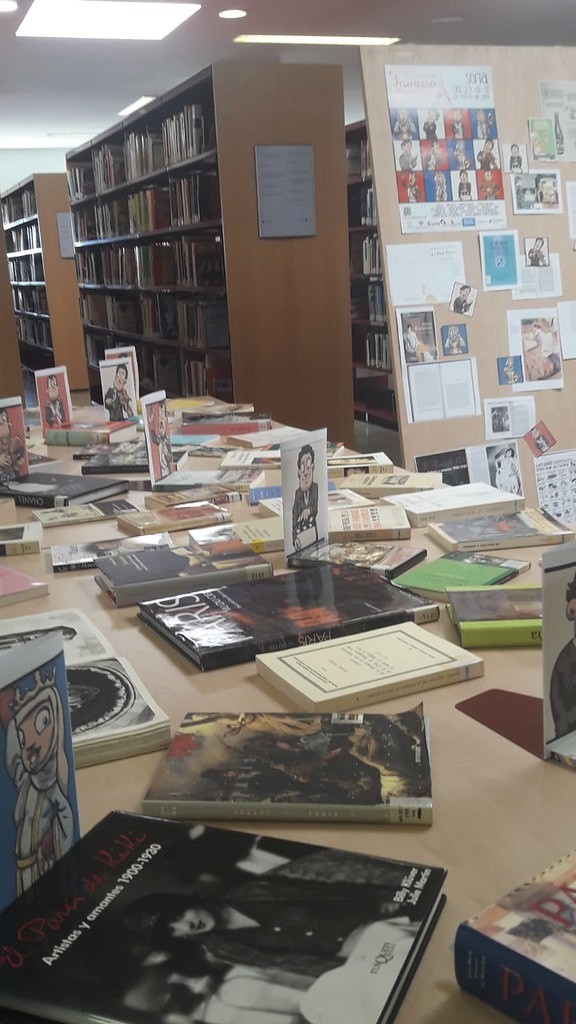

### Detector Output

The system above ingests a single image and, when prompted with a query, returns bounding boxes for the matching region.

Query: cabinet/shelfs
[0,59,398,454]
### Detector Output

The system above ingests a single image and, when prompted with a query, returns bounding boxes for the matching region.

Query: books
[1,103,394,400]
[0,403,576,1024]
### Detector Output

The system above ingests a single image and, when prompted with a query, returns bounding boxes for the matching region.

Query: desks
[0,395,575,1024]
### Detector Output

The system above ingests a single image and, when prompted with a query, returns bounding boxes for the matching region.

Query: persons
[404,325,419,355]
[494,448,519,494]
[122,872,422,977]
